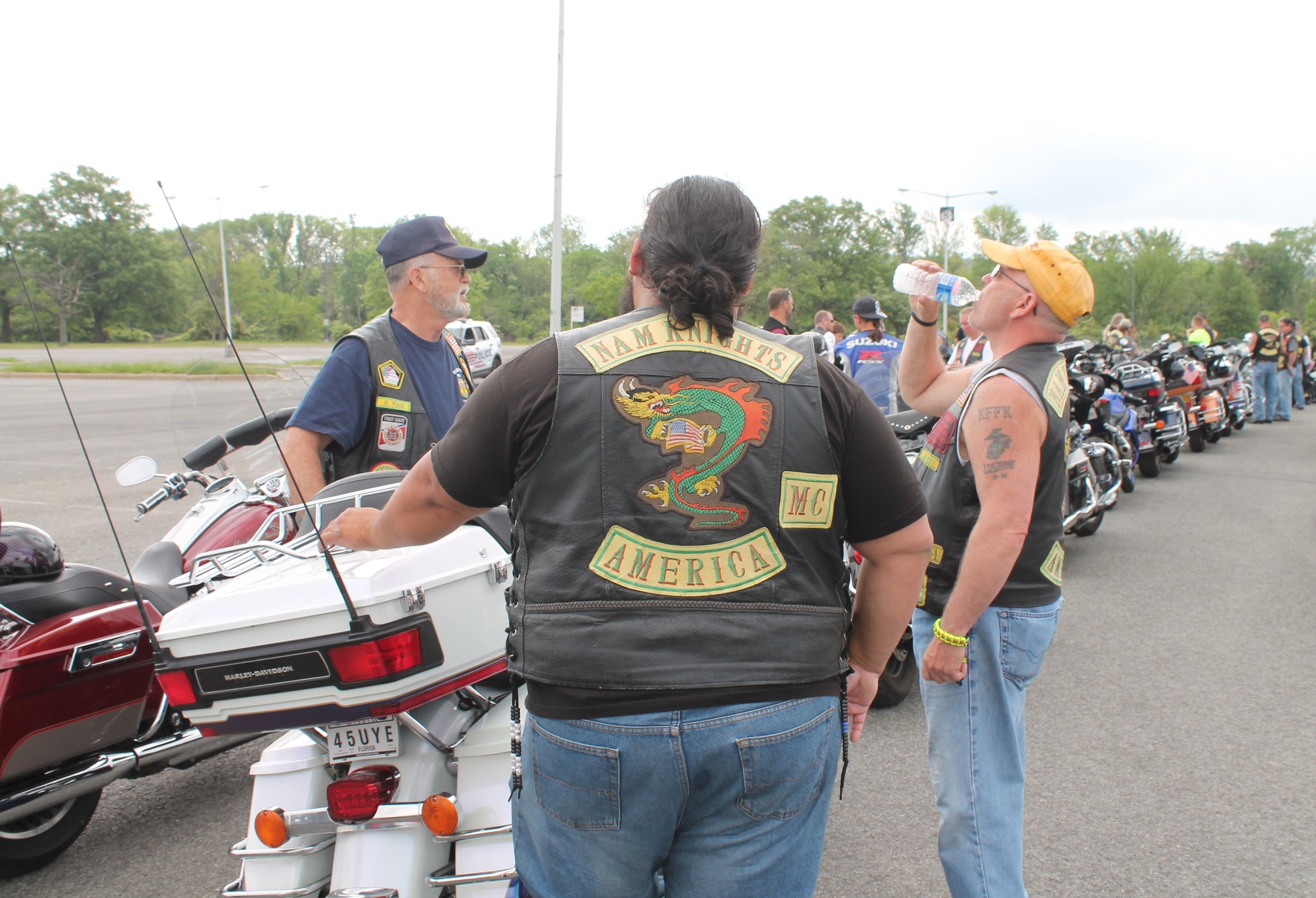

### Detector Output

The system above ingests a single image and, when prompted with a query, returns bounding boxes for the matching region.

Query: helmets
[0,522,64,580]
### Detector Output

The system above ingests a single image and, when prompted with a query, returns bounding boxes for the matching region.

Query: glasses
[410,264,465,285]
[821,319,834,323]
[783,290,792,305]
[990,264,1037,316]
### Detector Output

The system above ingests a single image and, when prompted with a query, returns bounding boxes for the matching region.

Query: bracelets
[932,618,969,647]
[911,309,937,326]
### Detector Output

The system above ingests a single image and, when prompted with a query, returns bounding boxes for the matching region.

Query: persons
[835,296,913,416]
[801,331,829,361]
[810,310,835,364]
[935,307,993,370]
[1103,313,1140,354]
[1274,316,1316,421]
[762,287,794,335]
[1247,315,1280,423]
[318,175,932,898]
[833,320,844,344]
[1186,313,1218,349]
[898,239,1094,898]
[280,216,488,518]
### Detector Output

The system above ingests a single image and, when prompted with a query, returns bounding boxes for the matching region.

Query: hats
[1260,314,1269,321]
[982,238,1095,327]
[375,217,488,270]
[852,296,888,319]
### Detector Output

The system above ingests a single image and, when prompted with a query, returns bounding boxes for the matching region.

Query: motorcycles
[13,177,524,898]
[833,326,1260,712]
[0,346,316,878]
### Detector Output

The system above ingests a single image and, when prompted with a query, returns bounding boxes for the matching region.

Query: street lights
[897,186,998,335]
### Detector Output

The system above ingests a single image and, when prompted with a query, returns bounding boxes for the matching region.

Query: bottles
[893,263,981,307]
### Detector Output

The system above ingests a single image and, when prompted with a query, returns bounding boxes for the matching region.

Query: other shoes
[1248,419,1264,424]
[1274,416,1289,422]
[1294,405,1303,410]
[1264,419,1272,423]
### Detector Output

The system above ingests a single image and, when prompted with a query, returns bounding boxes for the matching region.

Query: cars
[442,318,505,378]
